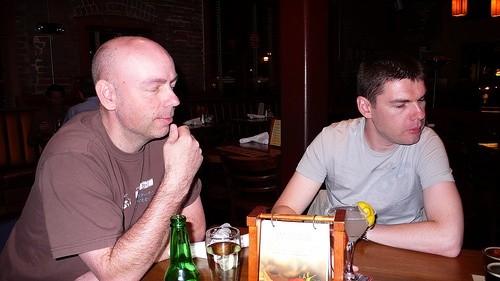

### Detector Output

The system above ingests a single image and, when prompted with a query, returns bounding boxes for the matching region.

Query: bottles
[164,215,201,281]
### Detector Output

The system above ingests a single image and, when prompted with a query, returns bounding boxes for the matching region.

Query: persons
[0,36,206,281]
[270,52,464,257]
[27,84,71,146]
[64,75,101,123]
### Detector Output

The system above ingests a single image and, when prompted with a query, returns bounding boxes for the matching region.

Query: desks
[214,140,280,195]
[141,227,486,281]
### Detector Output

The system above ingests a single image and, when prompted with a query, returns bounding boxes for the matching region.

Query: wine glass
[325,206,376,281]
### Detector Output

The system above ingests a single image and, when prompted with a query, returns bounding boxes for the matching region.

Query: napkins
[168,233,248,260]
[240,131,270,145]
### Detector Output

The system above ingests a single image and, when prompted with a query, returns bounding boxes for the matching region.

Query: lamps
[491,0,500,17]
[452,0,467,17]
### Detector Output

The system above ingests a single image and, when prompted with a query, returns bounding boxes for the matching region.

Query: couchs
[0,108,54,214]
[190,99,258,145]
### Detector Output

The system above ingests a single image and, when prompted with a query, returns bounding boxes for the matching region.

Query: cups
[485,263,500,281]
[484,247,500,271]
[206,227,241,281]
